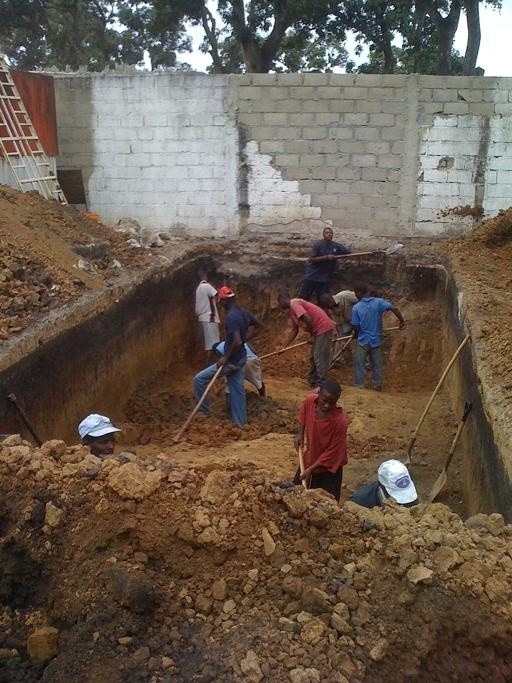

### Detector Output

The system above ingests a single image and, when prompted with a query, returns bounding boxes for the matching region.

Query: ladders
[0,58,68,206]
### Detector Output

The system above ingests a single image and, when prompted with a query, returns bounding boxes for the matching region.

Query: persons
[77,413,123,456]
[274,291,338,395]
[194,267,221,368]
[320,288,370,368]
[296,225,375,332]
[348,282,407,391]
[290,375,350,505]
[211,338,267,417]
[189,285,266,426]
[349,458,420,512]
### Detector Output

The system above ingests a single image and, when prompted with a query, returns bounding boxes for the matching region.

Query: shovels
[404,334,472,469]
[332,243,405,258]
[428,400,472,503]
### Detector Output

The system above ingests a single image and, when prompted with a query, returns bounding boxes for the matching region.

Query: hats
[217,285,236,301]
[78,412,121,440]
[376,458,419,506]
